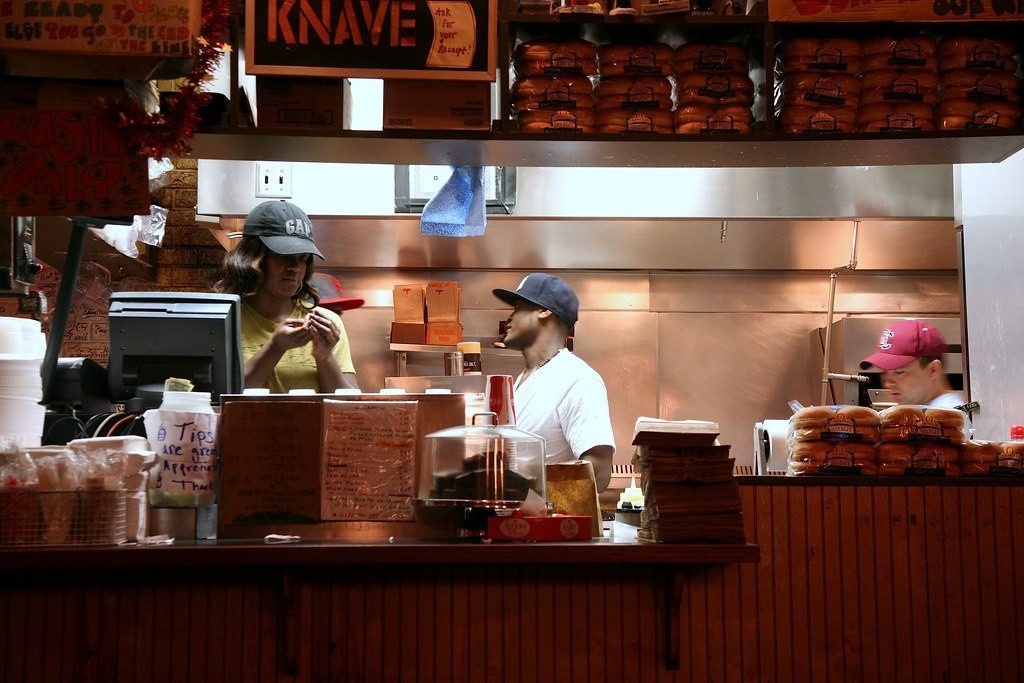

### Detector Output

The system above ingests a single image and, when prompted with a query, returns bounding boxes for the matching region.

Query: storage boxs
[256,74,352,132]
[149,505,218,540]
[391,321,426,345]
[382,78,490,130]
[425,321,462,345]
[0,489,127,548]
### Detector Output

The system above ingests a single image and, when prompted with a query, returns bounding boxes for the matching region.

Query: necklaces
[534,351,559,371]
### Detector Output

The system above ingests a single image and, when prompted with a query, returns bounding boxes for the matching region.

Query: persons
[860,318,971,420]
[223,202,358,393]
[492,272,616,493]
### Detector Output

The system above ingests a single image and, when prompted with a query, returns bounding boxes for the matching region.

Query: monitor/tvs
[107,292,244,405]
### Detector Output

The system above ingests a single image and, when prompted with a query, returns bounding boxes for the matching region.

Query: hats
[858,320,946,370]
[492,272,579,328]
[243,199,326,262]
[307,273,365,310]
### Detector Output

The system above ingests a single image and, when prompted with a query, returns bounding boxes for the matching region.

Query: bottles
[615,474,645,509]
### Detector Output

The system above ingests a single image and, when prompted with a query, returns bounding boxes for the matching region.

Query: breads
[789,407,1024,474]
[510,38,1021,129]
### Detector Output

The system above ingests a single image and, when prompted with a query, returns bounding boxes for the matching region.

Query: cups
[444,351,465,376]
[482,374,518,471]
